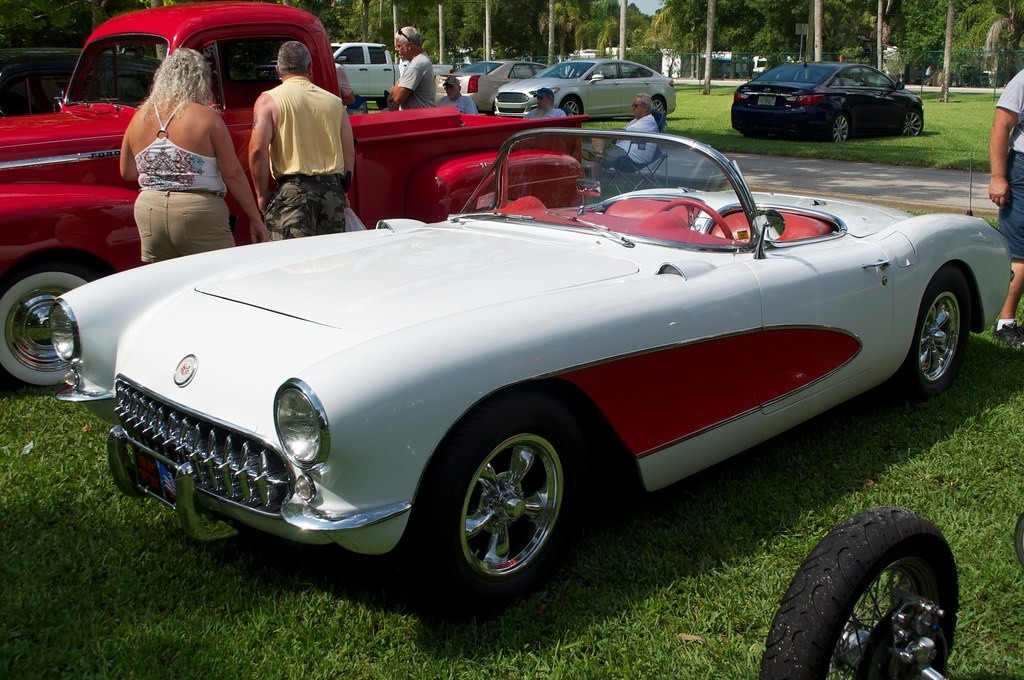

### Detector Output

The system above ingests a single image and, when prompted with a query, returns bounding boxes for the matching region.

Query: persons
[120,48,269,263]
[250,41,357,240]
[334,62,356,105]
[925,66,933,85]
[390,26,437,110]
[580,93,660,182]
[381,94,399,112]
[437,76,479,115]
[988,68,1024,348]
[523,88,566,119]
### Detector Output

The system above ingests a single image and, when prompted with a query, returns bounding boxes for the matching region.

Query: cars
[433,59,566,117]
[0,46,164,119]
[493,57,677,125]
[730,60,926,144]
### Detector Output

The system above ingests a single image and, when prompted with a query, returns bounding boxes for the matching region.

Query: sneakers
[992,322,1024,348]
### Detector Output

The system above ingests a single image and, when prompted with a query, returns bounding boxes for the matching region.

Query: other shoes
[582,148,606,164]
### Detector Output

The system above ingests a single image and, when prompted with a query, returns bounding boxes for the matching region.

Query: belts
[279,174,338,182]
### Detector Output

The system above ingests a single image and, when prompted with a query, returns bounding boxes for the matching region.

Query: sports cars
[46,123,1016,615]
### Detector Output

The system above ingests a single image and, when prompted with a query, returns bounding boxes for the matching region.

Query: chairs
[711,211,832,242]
[603,198,704,229]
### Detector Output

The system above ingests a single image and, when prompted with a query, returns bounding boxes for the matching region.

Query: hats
[533,88,554,98]
[443,77,460,85]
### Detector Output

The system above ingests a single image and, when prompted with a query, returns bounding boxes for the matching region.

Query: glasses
[632,104,641,108]
[536,96,544,100]
[446,84,454,89]
[398,27,410,43]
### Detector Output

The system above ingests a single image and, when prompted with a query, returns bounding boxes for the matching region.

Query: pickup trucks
[330,41,454,111]
[0,0,602,390]
